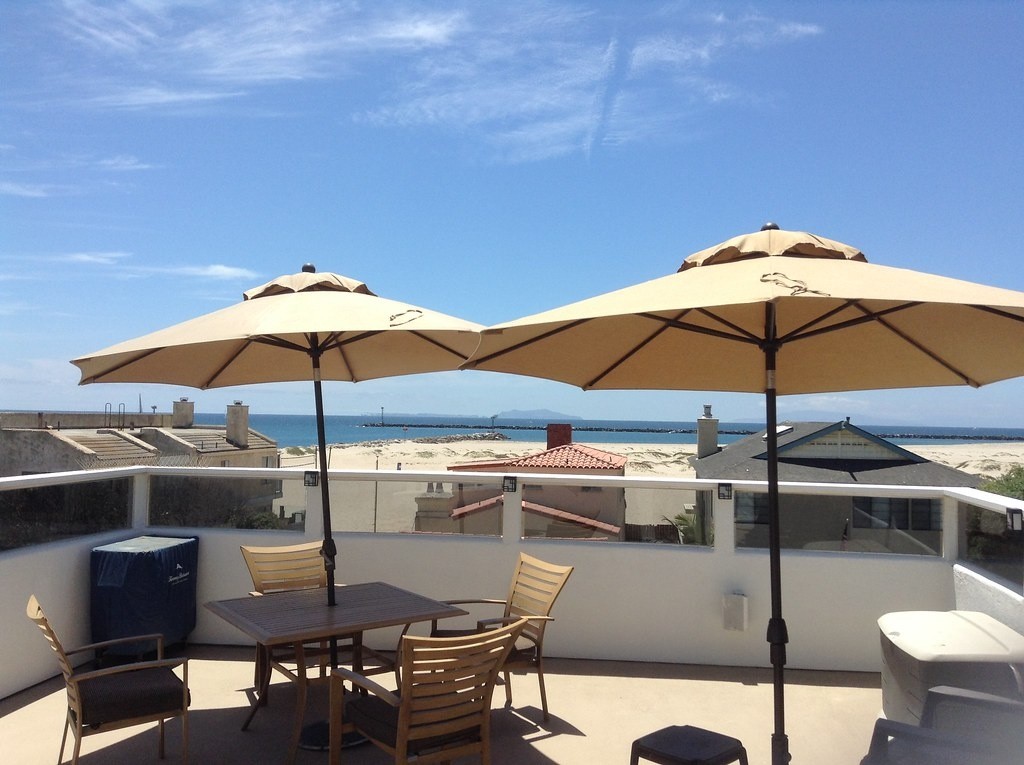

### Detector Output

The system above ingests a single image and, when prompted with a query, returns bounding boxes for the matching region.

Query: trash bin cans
[396,462,402,470]
[873,607,1024,734]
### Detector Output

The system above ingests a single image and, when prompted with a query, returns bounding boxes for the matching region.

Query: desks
[203,582,468,765]
[90,537,199,668]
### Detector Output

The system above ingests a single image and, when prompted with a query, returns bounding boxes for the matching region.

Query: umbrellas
[68,263,489,670]
[459,222,1024,765]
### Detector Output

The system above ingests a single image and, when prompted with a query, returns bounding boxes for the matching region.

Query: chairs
[429,552,574,721]
[328,618,530,765]
[240,541,358,705]
[26,594,189,765]
[860,685,1024,765]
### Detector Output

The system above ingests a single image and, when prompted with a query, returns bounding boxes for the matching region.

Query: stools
[629,725,753,765]
